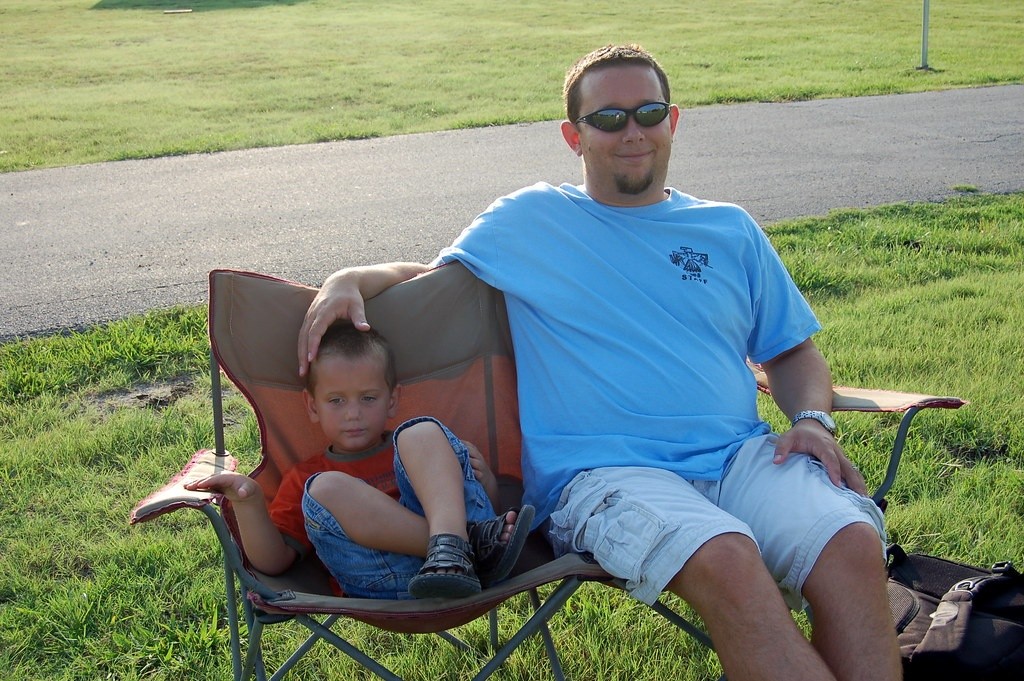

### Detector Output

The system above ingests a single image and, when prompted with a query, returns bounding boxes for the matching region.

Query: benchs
[130,259,967,679]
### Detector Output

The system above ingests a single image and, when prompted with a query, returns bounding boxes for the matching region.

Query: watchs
[792,410,836,437]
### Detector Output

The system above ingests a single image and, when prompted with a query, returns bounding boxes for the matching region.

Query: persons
[184,318,536,599]
[298,46,902,681]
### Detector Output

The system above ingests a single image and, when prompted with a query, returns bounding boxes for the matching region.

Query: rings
[852,465,856,470]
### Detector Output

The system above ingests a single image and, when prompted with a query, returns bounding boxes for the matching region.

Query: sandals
[408,533,481,600]
[466,504,536,582]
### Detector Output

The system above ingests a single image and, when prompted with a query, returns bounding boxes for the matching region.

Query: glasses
[575,102,670,132]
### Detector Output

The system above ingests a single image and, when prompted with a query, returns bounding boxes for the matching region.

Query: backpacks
[881,541,1024,681]
